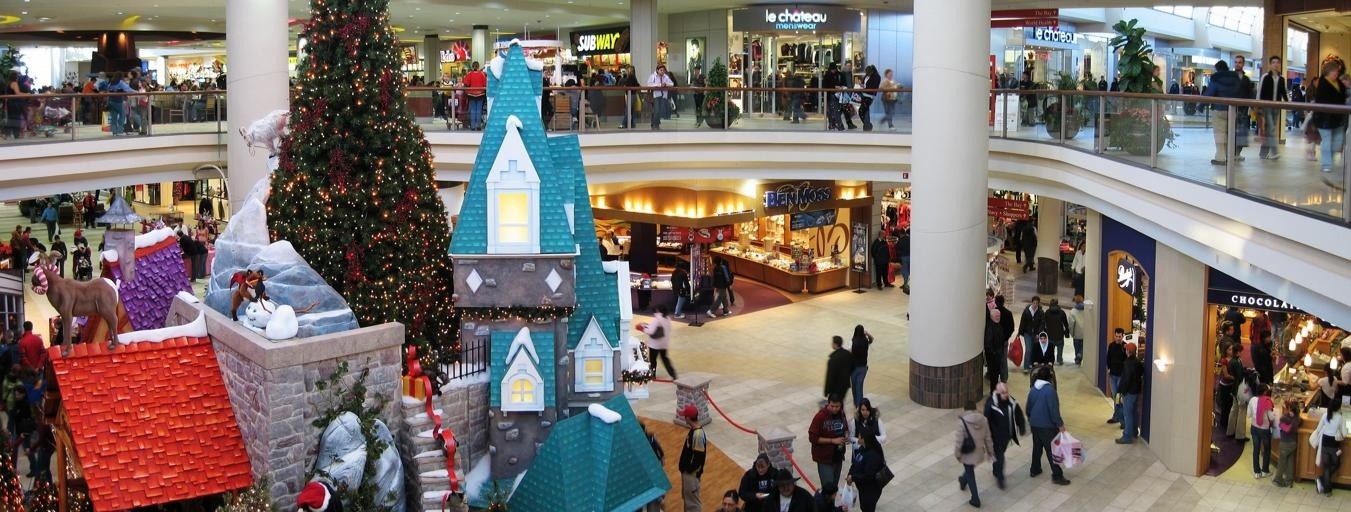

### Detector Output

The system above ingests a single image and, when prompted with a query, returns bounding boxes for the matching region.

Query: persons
[1115,342,1145,444]
[1218,306,1351,497]
[1104,327,1128,430]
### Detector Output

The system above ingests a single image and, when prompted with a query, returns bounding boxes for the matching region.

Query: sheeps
[238,109,291,158]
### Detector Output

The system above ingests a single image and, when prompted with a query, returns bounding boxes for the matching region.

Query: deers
[30,248,119,356]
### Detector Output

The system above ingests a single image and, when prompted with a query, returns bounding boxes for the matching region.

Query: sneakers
[1052,475,1069,485]
[706,310,715,317]
[1030,468,1042,476]
[674,312,685,319]
[724,310,731,315]
[1254,469,1290,487]
[969,499,979,507]
[1107,418,1138,443]
[957,475,966,490]
[1314,475,1322,493]
[878,283,905,291]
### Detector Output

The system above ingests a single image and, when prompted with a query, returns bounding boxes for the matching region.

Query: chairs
[51,83,218,125]
[576,101,602,131]
[552,94,574,132]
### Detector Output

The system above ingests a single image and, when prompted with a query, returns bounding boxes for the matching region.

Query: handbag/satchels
[1007,336,1024,367]
[1335,429,1344,441]
[957,414,975,453]
[874,464,894,489]
[1268,420,1274,433]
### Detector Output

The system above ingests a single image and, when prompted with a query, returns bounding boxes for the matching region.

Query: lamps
[1152,358,1169,372]
[1288,312,1339,371]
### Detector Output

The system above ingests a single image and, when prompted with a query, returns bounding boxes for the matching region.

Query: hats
[771,468,800,483]
[296,481,333,512]
[1123,342,1137,352]
[679,406,698,416]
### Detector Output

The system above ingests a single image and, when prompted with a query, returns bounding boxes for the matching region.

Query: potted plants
[1104,18,1180,155]
[698,55,742,129]
[1044,73,1086,141]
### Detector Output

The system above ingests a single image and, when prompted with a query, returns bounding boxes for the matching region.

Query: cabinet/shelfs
[593,234,851,314]
